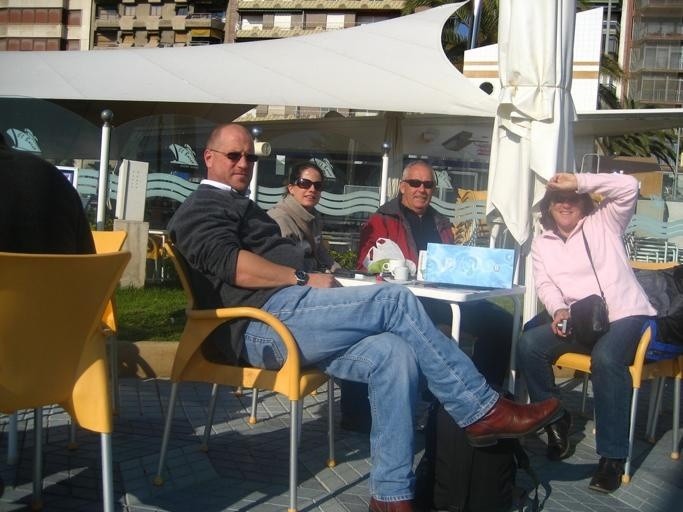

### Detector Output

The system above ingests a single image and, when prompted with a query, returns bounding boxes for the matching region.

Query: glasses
[210,149,259,161]
[402,180,435,188]
[294,179,324,190]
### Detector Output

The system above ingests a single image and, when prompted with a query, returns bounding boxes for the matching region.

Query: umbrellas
[486,0,582,332]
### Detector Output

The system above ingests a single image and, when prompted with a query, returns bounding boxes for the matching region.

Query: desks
[332,273,525,397]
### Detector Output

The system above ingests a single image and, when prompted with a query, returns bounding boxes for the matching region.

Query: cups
[354,272,364,279]
[391,266,409,282]
[382,259,405,276]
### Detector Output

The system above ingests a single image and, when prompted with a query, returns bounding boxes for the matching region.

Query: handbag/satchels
[571,295,609,342]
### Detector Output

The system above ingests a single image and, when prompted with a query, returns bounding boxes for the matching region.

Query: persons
[0,136,99,256]
[357,157,513,393]
[265,162,349,269]
[515,170,659,495]
[164,122,567,508]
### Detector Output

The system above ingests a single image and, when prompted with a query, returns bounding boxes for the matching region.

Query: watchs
[294,266,309,287]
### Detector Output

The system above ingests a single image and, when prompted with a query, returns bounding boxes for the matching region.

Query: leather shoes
[589,457,625,493]
[369,496,415,512]
[464,395,570,460]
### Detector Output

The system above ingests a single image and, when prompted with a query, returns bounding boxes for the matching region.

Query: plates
[379,276,419,286]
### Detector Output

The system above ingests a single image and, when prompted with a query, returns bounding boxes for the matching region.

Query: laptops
[57,165,79,191]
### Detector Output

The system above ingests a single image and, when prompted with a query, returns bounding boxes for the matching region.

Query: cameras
[556,319,568,334]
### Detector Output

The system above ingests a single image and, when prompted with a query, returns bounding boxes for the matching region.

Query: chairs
[0,251,131,512]
[552,260,683,483]
[91,230,129,415]
[154,244,335,511]
